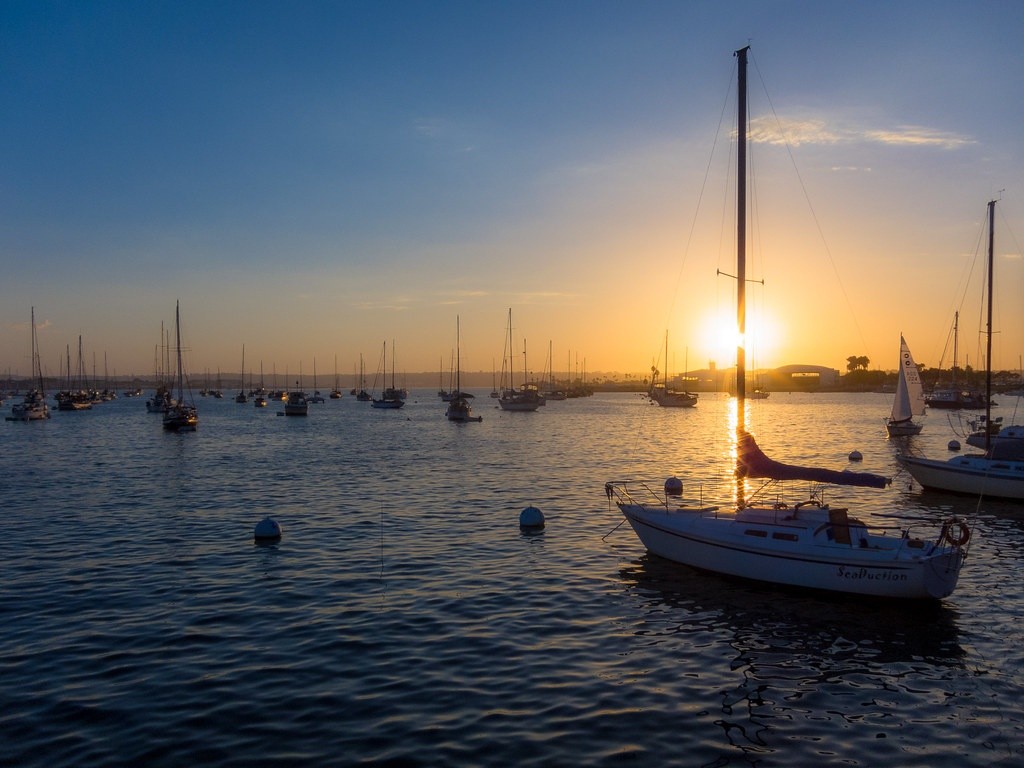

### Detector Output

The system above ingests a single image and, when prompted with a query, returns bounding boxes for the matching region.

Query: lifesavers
[942,517,970,546]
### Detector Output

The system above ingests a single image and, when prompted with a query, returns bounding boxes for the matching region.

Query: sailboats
[0,304,142,421]
[588,40,983,602]
[236,343,325,415]
[437,314,485,422]
[199,366,224,399]
[357,352,374,401]
[885,330,928,438]
[370,338,408,409]
[146,328,178,413]
[726,347,771,400]
[351,361,359,396]
[491,307,593,410]
[638,324,700,407]
[162,298,200,431]
[329,354,343,399]
[896,199,1024,501]
